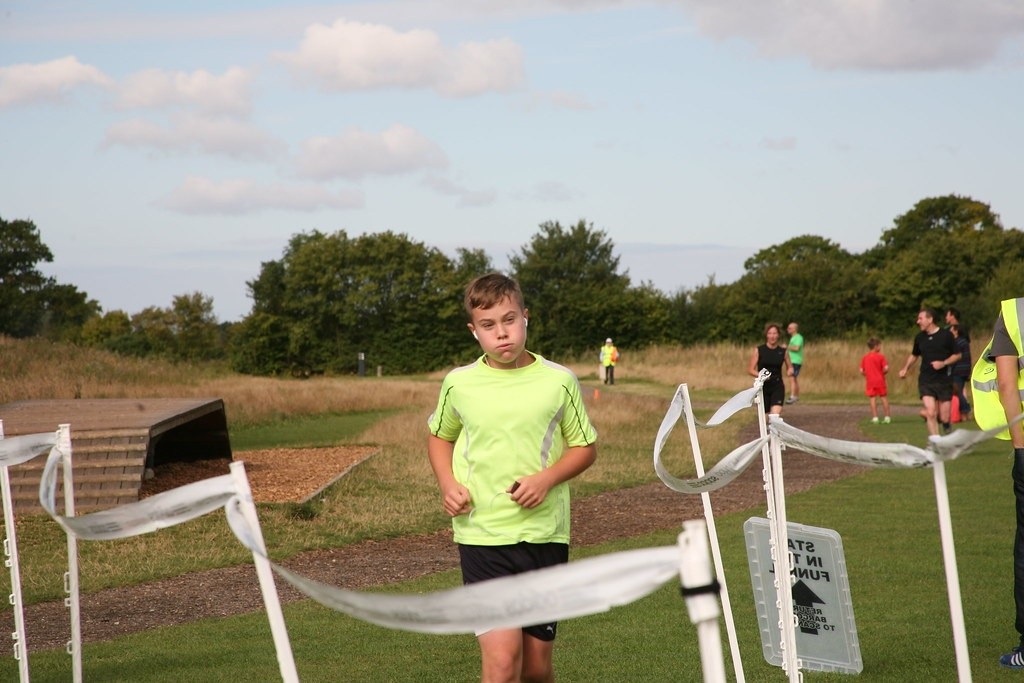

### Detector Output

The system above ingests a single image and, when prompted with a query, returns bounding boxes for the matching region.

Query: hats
[605,338,613,342]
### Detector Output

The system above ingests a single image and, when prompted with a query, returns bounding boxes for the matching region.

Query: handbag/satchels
[598,362,605,380]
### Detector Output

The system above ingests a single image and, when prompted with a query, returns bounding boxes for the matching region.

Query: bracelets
[943,361,947,366]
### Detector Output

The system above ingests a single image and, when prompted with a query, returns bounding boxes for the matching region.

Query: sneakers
[868,415,891,424]
[785,396,799,404]
[1000,646,1023,669]
[941,421,952,432]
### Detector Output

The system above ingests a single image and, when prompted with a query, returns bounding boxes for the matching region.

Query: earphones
[473,330,479,341]
[523,317,528,328]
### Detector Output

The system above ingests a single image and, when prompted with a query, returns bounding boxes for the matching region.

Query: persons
[428,272,599,683]
[781,321,804,404]
[750,322,795,425]
[898,309,964,445]
[970,297,1023,668]
[942,309,971,401]
[599,337,618,386]
[859,336,893,425]
[948,324,974,422]
